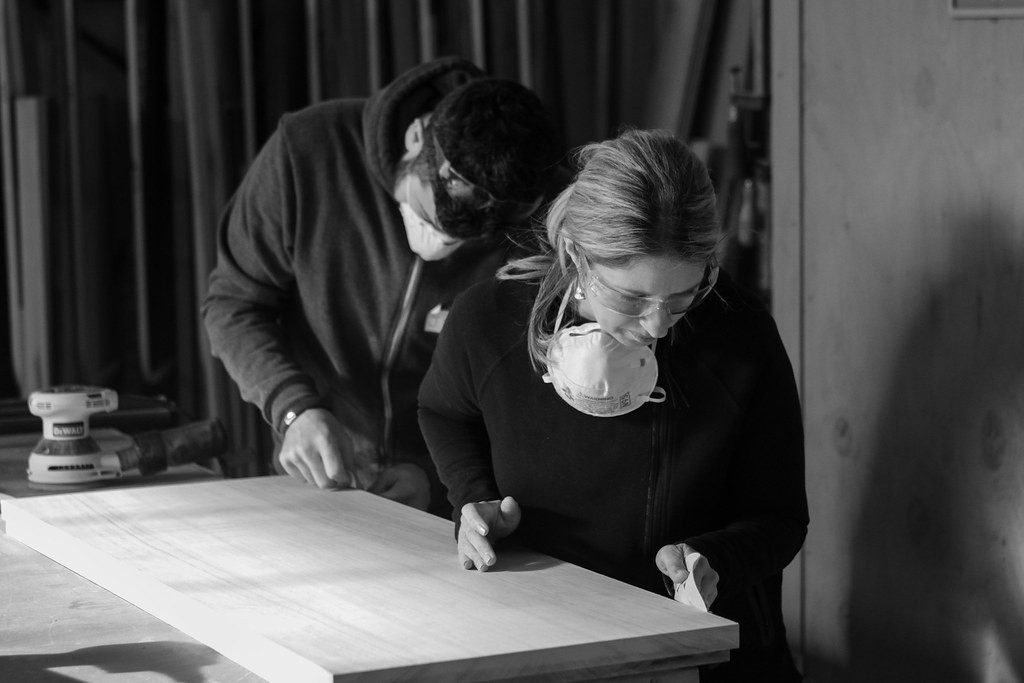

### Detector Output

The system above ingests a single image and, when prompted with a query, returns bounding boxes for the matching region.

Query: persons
[415,126,810,683]
[200,55,572,525]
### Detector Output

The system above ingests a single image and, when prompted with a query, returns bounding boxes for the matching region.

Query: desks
[0,451,731,683]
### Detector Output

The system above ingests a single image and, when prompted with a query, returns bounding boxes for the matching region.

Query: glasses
[432,135,544,220]
[581,254,719,318]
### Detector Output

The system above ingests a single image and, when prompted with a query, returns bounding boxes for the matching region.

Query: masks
[398,171,465,261]
[542,282,667,417]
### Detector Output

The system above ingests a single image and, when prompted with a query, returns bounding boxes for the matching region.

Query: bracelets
[279,401,339,433]
